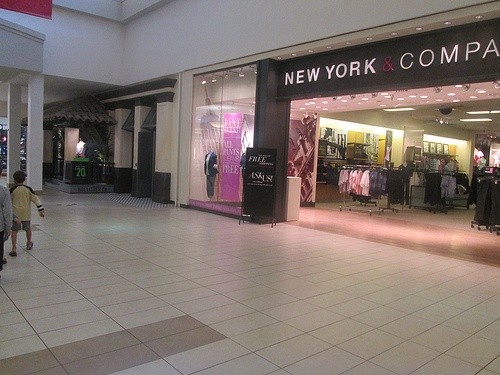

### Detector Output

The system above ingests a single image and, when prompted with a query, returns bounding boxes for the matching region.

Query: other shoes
[9,251,17,256]
[3,257,7,265]
[27,241,33,250]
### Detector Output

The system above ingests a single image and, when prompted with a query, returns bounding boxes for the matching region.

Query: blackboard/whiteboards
[243,147,278,217]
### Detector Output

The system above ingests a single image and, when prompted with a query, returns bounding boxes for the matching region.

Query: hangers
[327,153,500,185]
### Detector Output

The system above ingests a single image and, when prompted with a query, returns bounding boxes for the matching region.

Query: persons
[0,166,13,271]
[8,170,45,257]
[204,150,218,198]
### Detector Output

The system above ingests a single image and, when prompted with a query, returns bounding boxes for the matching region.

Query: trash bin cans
[97,162,114,183]
